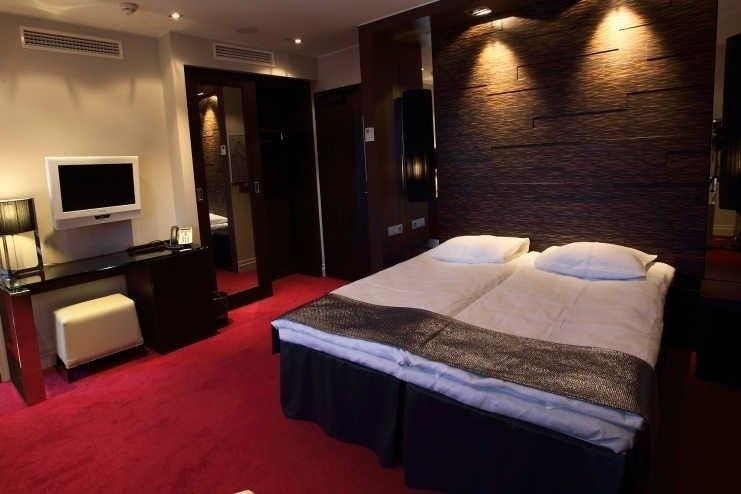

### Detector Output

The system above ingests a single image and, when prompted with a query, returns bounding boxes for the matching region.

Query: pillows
[534,241,659,280]
[431,234,531,264]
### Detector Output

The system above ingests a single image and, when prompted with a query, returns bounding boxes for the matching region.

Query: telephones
[170,226,193,247]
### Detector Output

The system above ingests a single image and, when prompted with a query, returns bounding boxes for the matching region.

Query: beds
[208,210,229,237]
[270,246,678,493]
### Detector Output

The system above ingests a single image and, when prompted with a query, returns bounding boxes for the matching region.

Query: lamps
[402,44,437,201]
[0,196,46,286]
[719,34,740,212]
[165,226,193,250]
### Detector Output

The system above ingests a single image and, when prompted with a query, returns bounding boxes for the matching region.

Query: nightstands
[698,279,740,387]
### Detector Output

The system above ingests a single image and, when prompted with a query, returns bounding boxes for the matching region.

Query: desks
[0,240,217,407]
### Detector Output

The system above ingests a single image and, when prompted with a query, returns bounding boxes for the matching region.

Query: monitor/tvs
[44,153,142,231]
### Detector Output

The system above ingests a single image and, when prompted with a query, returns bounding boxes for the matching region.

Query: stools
[51,289,145,384]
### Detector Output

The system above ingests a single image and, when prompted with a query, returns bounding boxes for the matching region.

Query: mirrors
[194,84,259,297]
